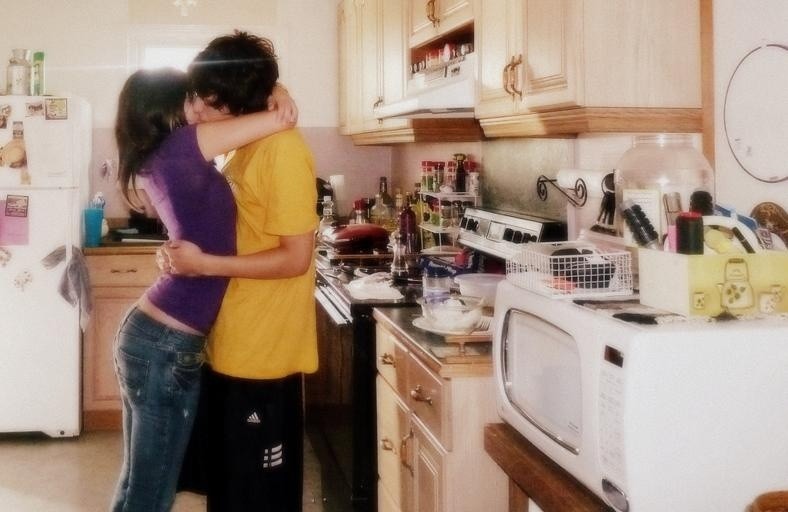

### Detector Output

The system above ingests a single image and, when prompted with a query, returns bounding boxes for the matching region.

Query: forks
[465,319,491,336]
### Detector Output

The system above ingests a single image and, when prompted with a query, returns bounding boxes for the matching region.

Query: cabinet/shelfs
[337,0,493,145]
[375,321,509,511]
[417,188,483,269]
[401,0,476,50]
[476,1,718,136]
[78,250,164,434]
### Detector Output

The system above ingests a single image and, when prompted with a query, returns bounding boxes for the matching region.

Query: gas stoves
[316,257,469,311]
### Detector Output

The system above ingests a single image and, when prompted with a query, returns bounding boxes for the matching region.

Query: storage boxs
[636,246,787,317]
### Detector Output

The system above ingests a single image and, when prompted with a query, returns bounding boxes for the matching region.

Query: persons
[155,27,320,512]
[108,63,298,512]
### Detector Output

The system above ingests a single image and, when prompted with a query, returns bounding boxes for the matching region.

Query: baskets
[506,241,633,301]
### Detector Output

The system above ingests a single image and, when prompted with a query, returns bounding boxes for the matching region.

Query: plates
[723,43,788,184]
[411,314,496,335]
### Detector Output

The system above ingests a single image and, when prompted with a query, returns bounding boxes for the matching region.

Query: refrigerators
[0,95,90,439]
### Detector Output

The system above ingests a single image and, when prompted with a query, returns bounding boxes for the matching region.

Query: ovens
[305,286,376,490]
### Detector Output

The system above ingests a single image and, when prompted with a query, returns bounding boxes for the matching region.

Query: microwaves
[494,275,788,512]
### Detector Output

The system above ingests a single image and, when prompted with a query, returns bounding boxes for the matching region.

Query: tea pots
[716,259,756,309]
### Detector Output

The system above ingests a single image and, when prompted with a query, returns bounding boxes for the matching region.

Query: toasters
[429,50,439,66]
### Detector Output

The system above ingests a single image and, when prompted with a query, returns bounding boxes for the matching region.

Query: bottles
[431,162,445,192]
[414,181,422,223]
[33,53,44,95]
[438,49,443,64]
[423,198,430,223]
[401,205,415,246]
[369,193,390,226]
[468,173,480,196]
[426,50,430,68]
[463,153,476,171]
[378,176,392,205]
[355,199,366,224]
[430,200,440,226]
[404,191,414,209]
[440,200,452,228]
[395,187,403,212]
[8,48,31,95]
[389,227,410,285]
[349,201,356,224]
[455,160,466,193]
[452,200,461,225]
[421,160,433,192]
[421,263,451,298]
[320,195,337,233]
[461,201,474,222]
[446,160,456,191]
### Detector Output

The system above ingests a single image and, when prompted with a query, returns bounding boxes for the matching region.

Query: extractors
[374,52,474,122]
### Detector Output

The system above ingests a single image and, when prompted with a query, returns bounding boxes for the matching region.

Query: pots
[320,226,389,250]
[129,206,163,234]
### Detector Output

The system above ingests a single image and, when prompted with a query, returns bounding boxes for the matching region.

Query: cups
[769,285,783,303]
[461,44,473,55]
[758,291,777,314]
[692,291,705,310]
[83,201,104,248]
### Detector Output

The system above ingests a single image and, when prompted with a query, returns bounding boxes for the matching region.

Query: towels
[41,244,95,333]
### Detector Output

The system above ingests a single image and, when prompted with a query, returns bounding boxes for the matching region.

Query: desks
[482,423,620,511]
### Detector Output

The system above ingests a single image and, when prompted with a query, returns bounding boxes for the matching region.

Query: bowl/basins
[454,272,507,307]
[414,295,483,331]
